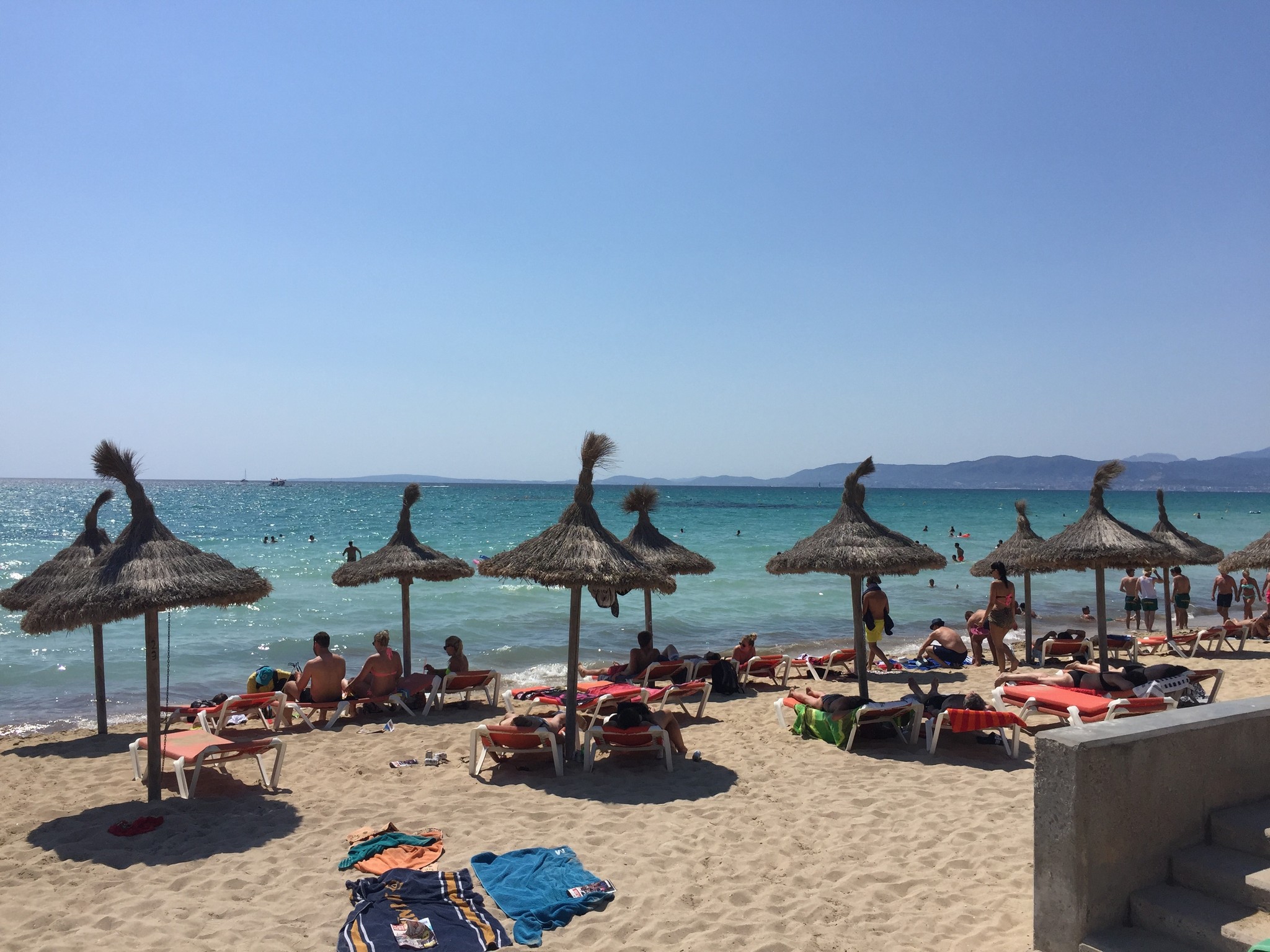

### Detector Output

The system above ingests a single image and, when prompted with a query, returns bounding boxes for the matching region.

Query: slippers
[977,732,1008,746]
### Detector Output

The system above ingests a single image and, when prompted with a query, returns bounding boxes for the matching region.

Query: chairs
[1140,627,1229,659]
[271,696,352,731]
[776,647,870,680]
[591,658,696,686]
[581,712,673,775]
[158,690,288,733]
[348,673,442,720]
[1032,636,1094,666]
[425,668,503,711]
[924,669,1225,759]
[1092,633,1138,665]
[738,653,794,689]
[128,726,286,800]
[693,657,743,684]
[468,722,565,779]
[780,696,930,751]
[1147,622,1251,653]
[647,677,716,720]
[502,682,650,715]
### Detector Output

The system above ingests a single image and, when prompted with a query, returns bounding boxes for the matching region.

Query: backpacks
[712,661,738,695]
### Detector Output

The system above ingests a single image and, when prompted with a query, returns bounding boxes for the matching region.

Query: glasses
[989,570,994,574]
[443,646,454,650]
[739,642,746,648]
[372,642,374,646]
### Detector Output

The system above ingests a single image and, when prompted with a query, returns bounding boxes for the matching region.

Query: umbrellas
[0,441,275,806]
[477,433,676,762]
[331,483,475,677]
[968,501,1063,665]
[615,481,719,650]
[1144,486,1225,639]
[1217,531,1270,573]
[764,456,949,698]
[1043,462,1180,675]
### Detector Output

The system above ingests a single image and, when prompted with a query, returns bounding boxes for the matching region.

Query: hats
[930,618,944,630]
[256,667,273,685]
[1142,567,1155,574]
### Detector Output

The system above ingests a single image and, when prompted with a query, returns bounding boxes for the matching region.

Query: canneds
[570,750,583,762]
[425,750,433,758]
[1020,659,1025,665]
[692,750,701,761]
[424,758,437,765]
[434,752,447,759]
[433,756,440,765]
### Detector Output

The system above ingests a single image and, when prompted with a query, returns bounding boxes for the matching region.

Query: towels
[795,652,824,666]
[345,821,444,876]
[512,686,604,707]
[339,832,436,869]
[945,708,1028,733]
[336,868,513,952]
[586,676,706,702]
[792,703,911,747]
[469,845,616,948]
[1132,670,1206,699]
[597,674,639,682]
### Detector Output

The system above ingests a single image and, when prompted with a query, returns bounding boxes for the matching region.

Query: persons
[862,561,1039,674]
[1031,629,1086,651]
[1081,566,1190,632]
[994,659,1191,692]
[246,629,469,726]
[342,541,362,563]
[1197,512,1201,519]
[786,676,995,711]
[264,534,283,544]
[680,528,782,555]
[578,631,757,678]
[915,515,1006,561]
[1211,570,1270,637]
[494,701,688,754]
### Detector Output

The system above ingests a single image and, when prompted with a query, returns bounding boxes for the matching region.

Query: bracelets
[1076,630,1078,635]
